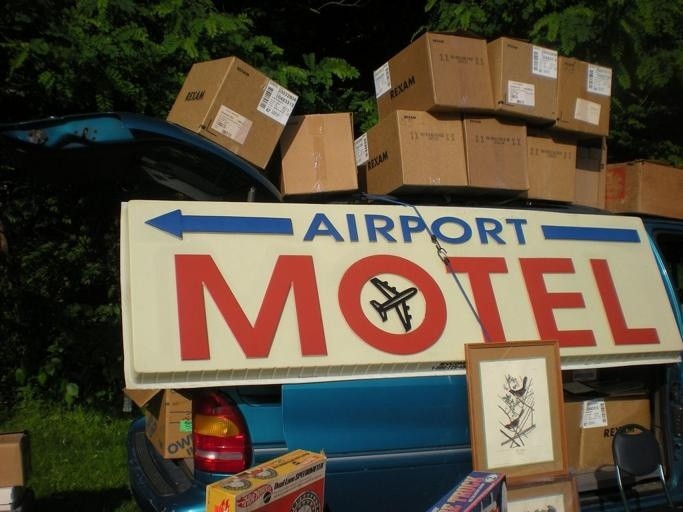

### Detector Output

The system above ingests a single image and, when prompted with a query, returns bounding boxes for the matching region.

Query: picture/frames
[507,475,581,512]
[464,339,569,483]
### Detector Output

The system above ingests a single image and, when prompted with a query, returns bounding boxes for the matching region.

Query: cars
[0,111,683,512]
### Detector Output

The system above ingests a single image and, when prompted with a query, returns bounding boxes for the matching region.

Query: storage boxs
[517,127,577,208]
[573,137,611,213]
[277,111,359,200]
[487,37,560,126]
[426,471,507,512]
[121,388,193,459]
[565,398,651,470]
[166,57,298,170]
[462,114,530,205]
[206,449,327,512]
[608,158,683,221]
[0,431,32,487]
[374,33,495,121]
[551,53,614,138]
[355,110,469,204]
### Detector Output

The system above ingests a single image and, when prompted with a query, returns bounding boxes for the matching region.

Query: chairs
[612,423,676,512]
